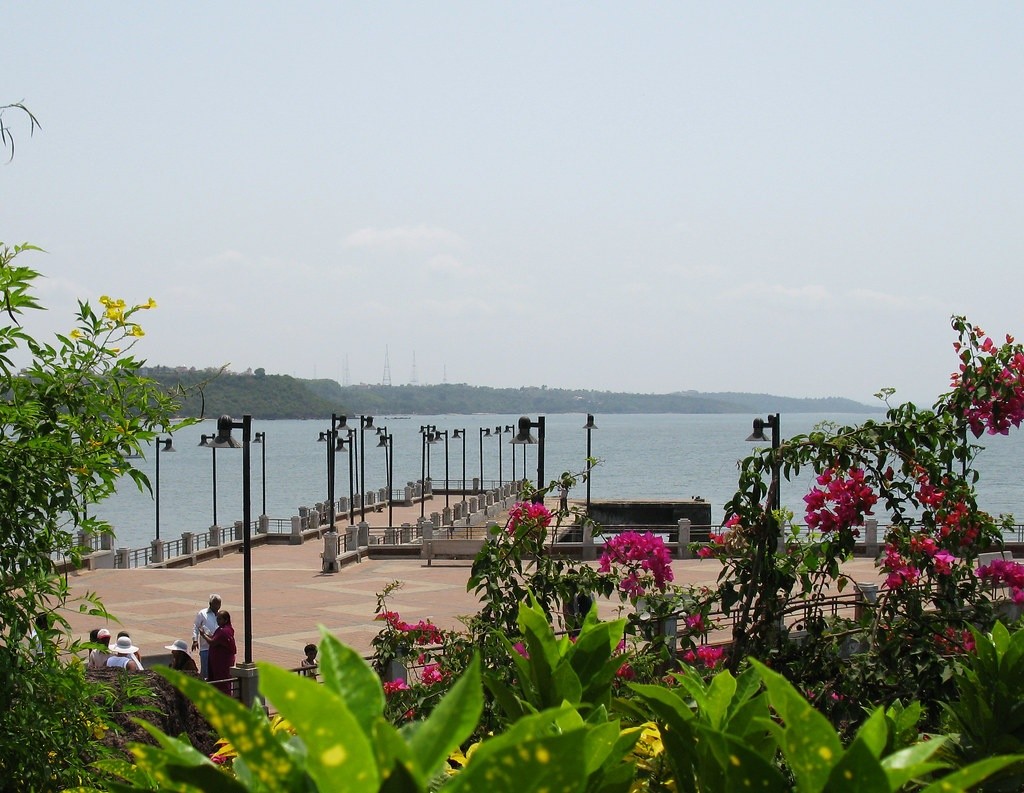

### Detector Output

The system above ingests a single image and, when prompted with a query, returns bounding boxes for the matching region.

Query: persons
[164,639,199,675]
[301,644,319,684]
[192,593,224,680]
[88,629,143,674]
[557,485,569,517]
[198,610,236,695]
[29,612,48,658]
[563,568,592,641]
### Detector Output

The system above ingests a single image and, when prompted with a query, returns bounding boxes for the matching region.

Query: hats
[165,639,192,657]
[108,637,140,654]
[97,629,111,639]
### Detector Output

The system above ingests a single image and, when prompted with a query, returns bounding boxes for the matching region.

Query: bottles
[202,625,212,635]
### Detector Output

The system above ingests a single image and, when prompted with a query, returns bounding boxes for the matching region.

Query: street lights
[745,412,780,512]
[156,437,176,539]
[508,416,546,505]
[197,433,217,525]
[252,432,265,514]
[583,412,599,520]
[316,413,527,532]
[204,415,252,662]
